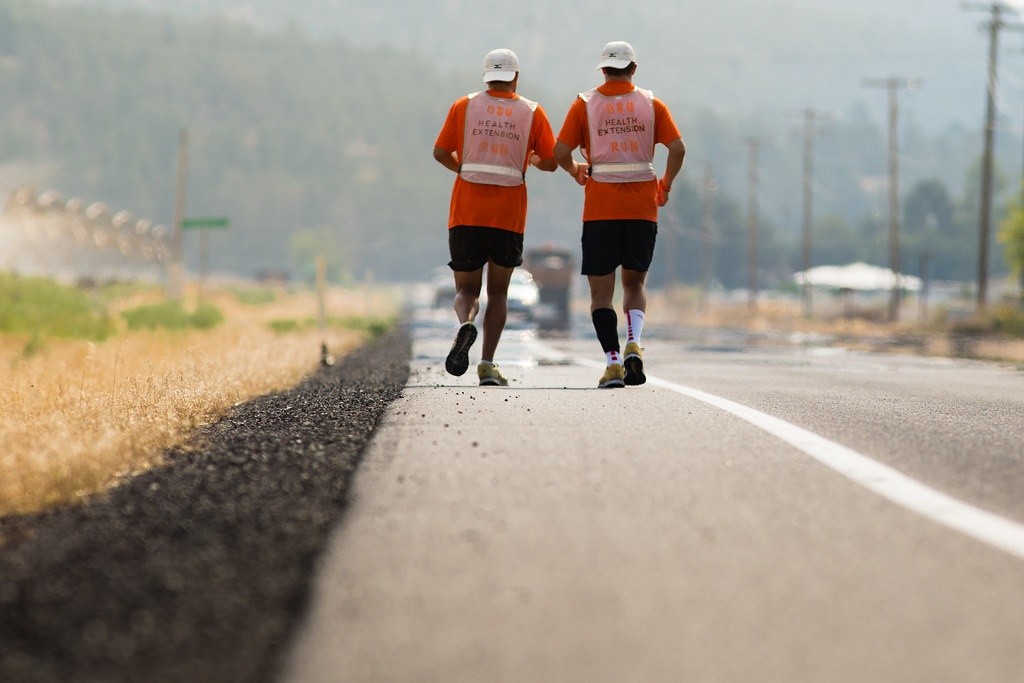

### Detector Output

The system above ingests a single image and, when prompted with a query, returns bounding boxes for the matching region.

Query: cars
[479,267,539,321]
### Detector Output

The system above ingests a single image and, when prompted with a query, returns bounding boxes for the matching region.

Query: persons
[431,48,559,387]
[550,40,687,389]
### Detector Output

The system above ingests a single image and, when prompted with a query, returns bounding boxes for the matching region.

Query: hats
[483,49,519,83]
[597,41,637,70]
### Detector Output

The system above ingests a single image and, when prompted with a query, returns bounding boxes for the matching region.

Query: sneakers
[623,343,646,386]
[598,364,625,387]
[445,322,478,377]
[477,364,508,386]
